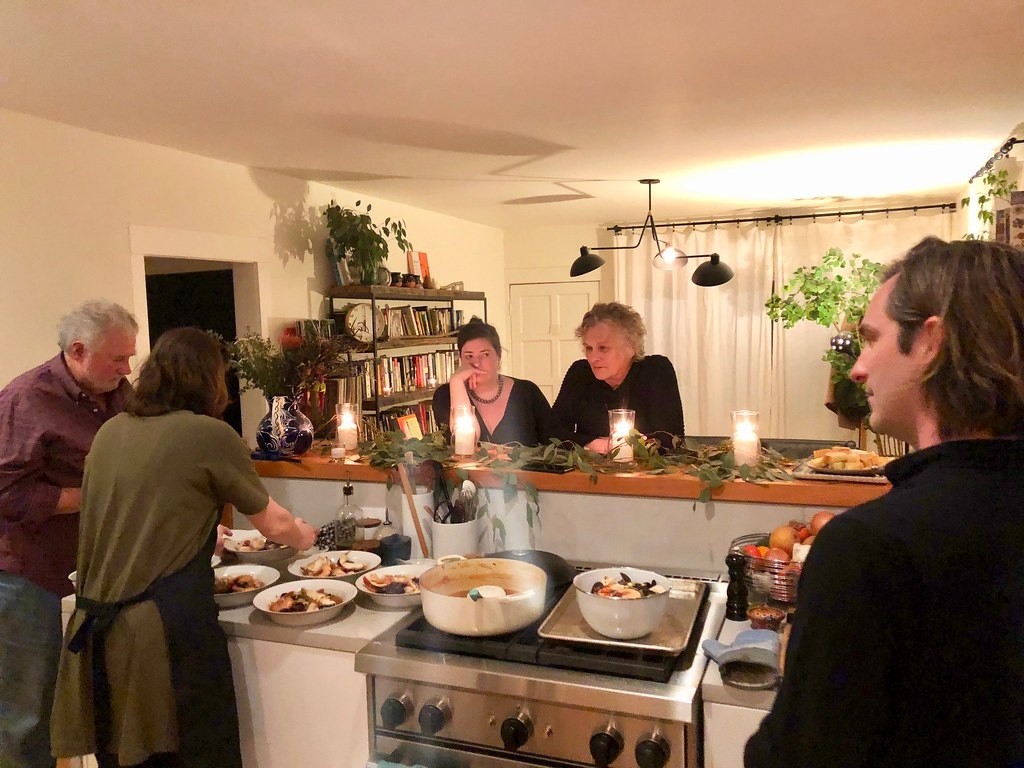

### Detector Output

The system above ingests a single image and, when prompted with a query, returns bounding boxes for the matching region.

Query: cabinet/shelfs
[328,284,488,448]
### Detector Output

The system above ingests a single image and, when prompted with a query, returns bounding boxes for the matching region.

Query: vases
[255,395,314,454]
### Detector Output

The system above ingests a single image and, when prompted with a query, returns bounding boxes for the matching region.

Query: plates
[804,458,894,474]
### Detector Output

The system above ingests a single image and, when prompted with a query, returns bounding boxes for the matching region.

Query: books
[407,252,430,283]
[327,238,352,286]
[326,304,463,441]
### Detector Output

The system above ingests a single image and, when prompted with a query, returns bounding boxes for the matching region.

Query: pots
[418,552,548,638]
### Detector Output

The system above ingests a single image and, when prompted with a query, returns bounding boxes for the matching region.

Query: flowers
[232,319,355,395]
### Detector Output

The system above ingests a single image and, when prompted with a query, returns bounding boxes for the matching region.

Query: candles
[456,406,474,456]
[611,411,634,461]
[338,411,359,451]
[733,417,757,466]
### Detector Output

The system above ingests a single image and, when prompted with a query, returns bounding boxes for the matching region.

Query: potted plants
[324,200,414,282]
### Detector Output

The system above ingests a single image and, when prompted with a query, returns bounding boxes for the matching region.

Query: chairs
[860,412,903,458]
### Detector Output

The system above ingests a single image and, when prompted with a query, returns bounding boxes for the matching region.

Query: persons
[431,319,552,447]
[0,297,136,768]
[50,327,316,768]
[539,304,684,457]
[744,239,1024,768]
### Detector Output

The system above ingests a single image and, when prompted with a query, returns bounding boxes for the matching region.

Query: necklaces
[466,375,503,403]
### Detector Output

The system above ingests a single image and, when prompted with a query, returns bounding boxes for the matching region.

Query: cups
[401,490,434,559]
[453,405,476,460]
[330,442,346,462]
[431,515,480,560]
[731,409,761,470]
[335,402,360,452]
[608,409,635,465]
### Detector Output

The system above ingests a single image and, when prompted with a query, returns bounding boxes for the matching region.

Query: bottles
[334,485,364,549]
[373,521,401,541]
[380,532,412,566]
[356,518,382,541]
[389,272,420,289]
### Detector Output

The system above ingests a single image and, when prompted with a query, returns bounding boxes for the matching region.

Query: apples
[742,512,837,597]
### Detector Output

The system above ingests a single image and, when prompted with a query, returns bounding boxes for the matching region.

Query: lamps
[570,179,734,289]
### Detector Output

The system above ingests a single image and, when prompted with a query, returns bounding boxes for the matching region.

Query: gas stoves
[351,563,732,768]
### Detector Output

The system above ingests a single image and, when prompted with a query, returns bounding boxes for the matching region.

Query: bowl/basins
[572,567,673,640]
[355,564,432,609]
[287,550,381,580]
[729,530,806,602]
[213,563,280,607]
[253,578,358,626]
[226,529,299,565]
[747,605,785,630]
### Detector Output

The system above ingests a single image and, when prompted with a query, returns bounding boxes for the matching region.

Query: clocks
[345,303,386,342]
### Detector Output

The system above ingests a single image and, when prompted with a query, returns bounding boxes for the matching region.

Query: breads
[810,446,888,470]
